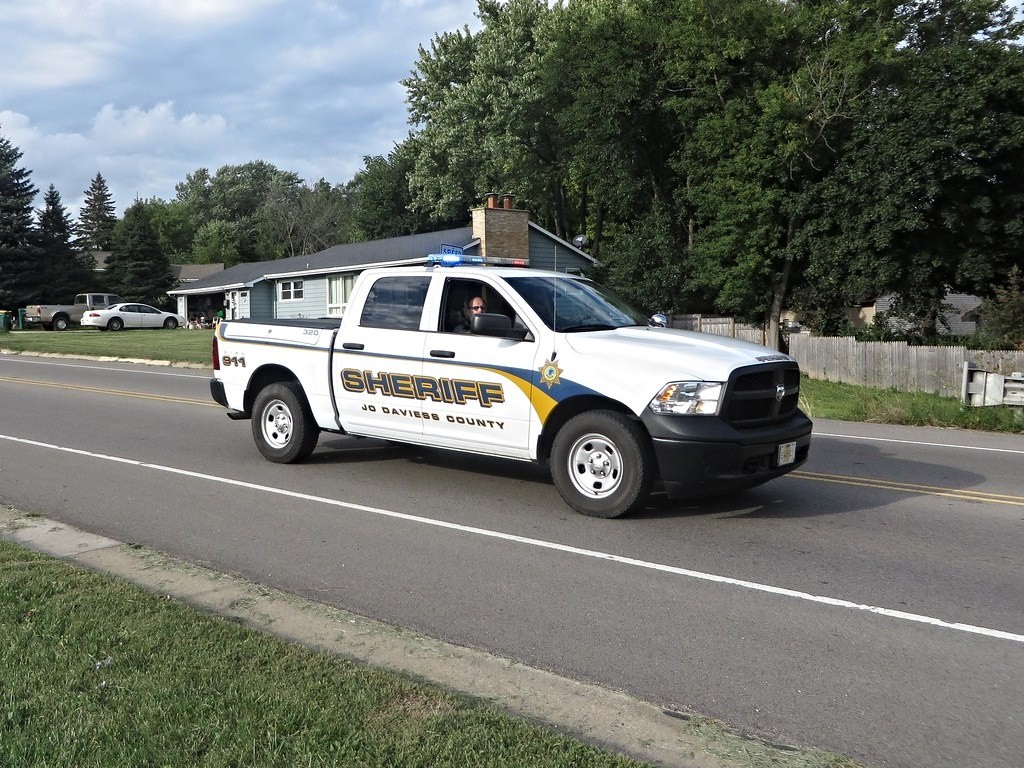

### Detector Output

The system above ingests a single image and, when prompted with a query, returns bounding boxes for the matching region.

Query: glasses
[468,306,486,311]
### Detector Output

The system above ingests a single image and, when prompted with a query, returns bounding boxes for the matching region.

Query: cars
[81,303,186,331]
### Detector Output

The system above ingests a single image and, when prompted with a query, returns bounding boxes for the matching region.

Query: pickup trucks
[210,254,814,519]
[24,293,127,331]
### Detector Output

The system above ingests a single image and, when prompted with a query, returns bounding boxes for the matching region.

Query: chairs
[212,316,223,329]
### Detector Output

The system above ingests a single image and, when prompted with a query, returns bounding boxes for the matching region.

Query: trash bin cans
[18,308,27,330]
[0,310,12,331]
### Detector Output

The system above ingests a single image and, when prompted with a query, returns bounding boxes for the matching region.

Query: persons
[535,293,577,327]
[453,296,486,334]
